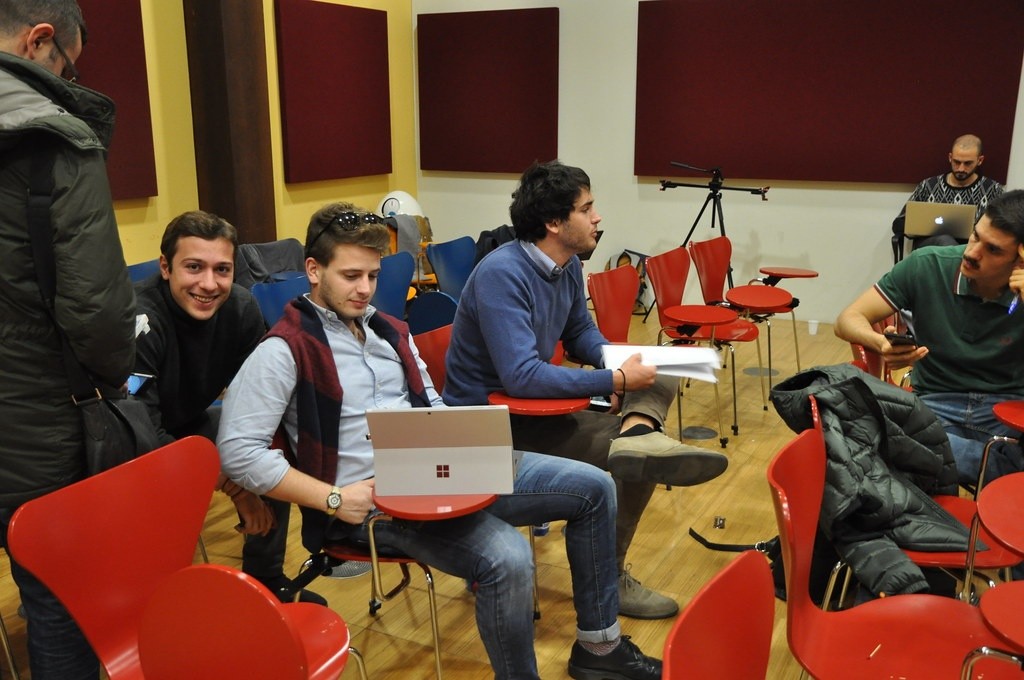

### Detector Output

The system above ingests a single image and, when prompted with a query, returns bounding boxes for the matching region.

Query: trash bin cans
[374,191,424,218]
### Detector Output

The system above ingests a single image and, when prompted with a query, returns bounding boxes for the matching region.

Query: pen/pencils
[1008,292,1021,315]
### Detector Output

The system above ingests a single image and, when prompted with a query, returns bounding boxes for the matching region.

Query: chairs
[140,238,1024,680]
[7,438,219,680]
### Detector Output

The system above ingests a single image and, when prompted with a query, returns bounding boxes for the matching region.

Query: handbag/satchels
[71,385,163,478]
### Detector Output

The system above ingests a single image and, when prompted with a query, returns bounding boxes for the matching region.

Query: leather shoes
[568,633,663,680]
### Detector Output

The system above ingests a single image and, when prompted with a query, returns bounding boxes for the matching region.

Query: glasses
[306,211,381,256]
[29,23,79,83]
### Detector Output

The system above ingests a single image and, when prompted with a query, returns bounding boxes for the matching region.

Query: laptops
[904,201,977,240]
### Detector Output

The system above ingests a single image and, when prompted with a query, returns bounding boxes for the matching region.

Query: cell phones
[884,333,918,350]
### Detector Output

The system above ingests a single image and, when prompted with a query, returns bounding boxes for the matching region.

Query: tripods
[640,161,770,322]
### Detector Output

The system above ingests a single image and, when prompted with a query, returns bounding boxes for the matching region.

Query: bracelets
[613,368,626,401]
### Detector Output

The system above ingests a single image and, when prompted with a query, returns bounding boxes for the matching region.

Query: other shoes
[272,578,329,608]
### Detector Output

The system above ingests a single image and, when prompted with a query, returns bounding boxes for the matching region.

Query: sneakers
[617,563,680,619]
[607,427,728,487]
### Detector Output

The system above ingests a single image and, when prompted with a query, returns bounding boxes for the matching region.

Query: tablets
[367,406,518,480]
[126,371,156,397]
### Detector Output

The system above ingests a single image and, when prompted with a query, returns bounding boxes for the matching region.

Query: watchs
[326,486,343,515]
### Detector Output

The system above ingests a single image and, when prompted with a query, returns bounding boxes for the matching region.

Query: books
[601,344,721,384]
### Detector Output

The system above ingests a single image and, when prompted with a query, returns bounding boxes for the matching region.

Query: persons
[441,163,729,619]
[835,190,1024,489]
[892,134,1005,248]
[216,201,664,680]
[0,0,132,680]
[132,210,327,607]
